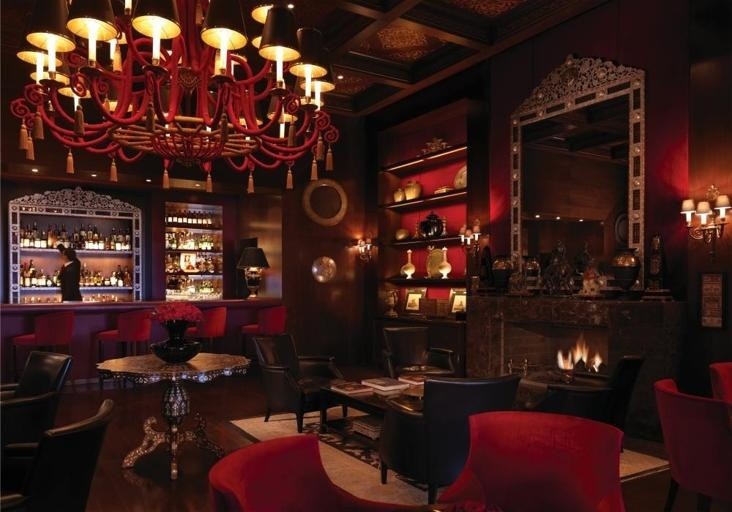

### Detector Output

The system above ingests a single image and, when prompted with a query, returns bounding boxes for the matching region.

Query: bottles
[20,259,132,287]
[167,275,215,293]
[164,253,214,273]
[18,222,132,250]
[165,209,217,229]
[22,294,118,304]
[164,232,220,250]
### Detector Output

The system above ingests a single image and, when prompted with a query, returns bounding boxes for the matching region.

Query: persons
[54,238,81,301]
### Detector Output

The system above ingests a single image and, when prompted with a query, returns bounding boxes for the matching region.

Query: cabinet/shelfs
[378,142,467,330]
[8,186,144,304]
[164,200,223,301]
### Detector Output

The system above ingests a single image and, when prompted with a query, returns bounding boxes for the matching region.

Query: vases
[393,180,421,202]
[150,320,201,363]
[609,247,640,301]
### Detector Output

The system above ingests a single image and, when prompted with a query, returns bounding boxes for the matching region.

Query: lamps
[12,0,340,195]
[680,195,732,264]
[349,233,374,271]
[236,246,270,300]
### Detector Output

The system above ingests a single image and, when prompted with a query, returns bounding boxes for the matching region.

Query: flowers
[147,301,204,325]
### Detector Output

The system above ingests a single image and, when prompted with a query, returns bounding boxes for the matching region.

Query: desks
[317,382,422,453]
[97,354,255,481]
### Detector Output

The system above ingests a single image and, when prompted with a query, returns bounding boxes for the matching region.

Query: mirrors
[508,54,645,299]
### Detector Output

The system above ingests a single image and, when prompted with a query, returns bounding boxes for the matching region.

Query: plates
[425,248,445,278]
[453,165,467,189]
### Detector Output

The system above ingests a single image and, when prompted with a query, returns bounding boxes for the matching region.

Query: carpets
[224,404,672,509]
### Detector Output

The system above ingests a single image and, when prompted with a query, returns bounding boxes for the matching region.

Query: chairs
[250,333,348,434]
[653,379,732,511]
[708,362,732,401]
[0,350,73,446]
[94,309,153,392]
[432,410,625,512]
[10,309,77,394]
[208,434,424,512]
[240,305,288,358]
[377,372,522,505]
[532,354,641,454]
[188,307,227,353]
[0,398,115,512]
